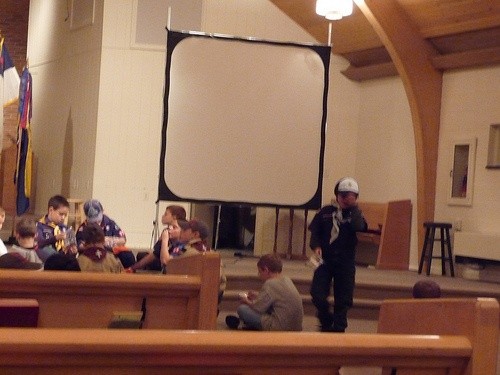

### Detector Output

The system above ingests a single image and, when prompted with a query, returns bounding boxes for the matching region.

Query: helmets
[334,177,359,196]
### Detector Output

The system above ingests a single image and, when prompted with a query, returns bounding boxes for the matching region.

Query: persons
[0,195,226,316]
[310,178,368,332]
[225,253,303,331]
[412,280,441,299]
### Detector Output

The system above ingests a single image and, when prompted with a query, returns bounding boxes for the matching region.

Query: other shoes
[225,316,248,331]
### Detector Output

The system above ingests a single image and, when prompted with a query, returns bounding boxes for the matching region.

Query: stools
[417,222,455,278]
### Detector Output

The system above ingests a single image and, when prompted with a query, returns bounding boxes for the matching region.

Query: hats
[76,222,104,249]
[83,200,103,223]
[177,219,209,240]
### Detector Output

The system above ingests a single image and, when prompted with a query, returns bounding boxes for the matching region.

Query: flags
[0,35,33,217]
